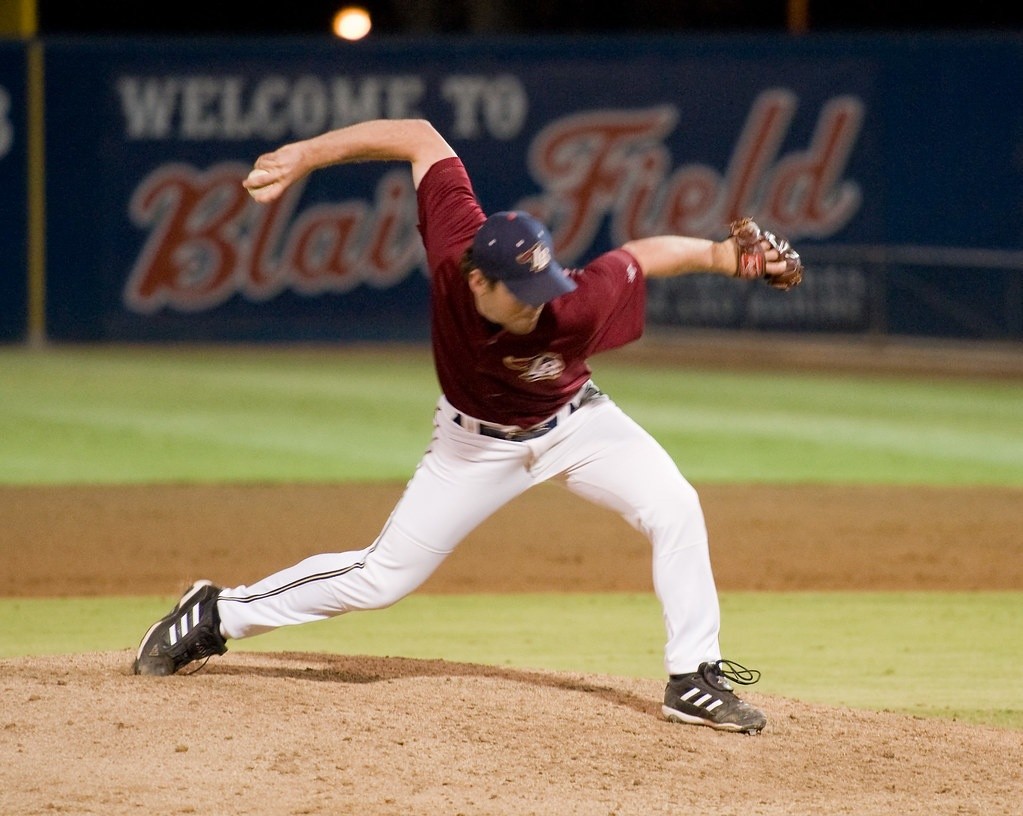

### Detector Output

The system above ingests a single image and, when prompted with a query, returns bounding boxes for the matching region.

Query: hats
[472,210,578,307]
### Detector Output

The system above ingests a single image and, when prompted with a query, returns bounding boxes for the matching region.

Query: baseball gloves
[730,214,805,294]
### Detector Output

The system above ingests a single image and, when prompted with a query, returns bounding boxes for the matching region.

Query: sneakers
[661,659,767,733]
[135,580,228,675]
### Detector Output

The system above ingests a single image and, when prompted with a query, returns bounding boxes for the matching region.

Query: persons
[132,117,805,731]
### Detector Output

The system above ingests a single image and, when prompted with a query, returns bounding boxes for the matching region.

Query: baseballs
[248,168,272,197]
